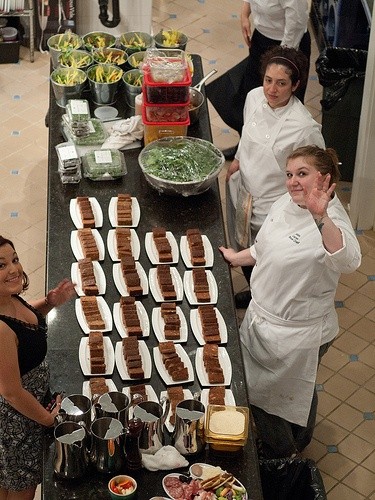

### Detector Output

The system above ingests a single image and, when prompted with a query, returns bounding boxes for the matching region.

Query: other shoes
[235,291,252,308]
[222,142,240,162]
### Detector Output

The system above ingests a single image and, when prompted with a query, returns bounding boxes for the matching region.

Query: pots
[187,69,218,127]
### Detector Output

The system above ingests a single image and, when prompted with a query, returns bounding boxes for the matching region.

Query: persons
[226,46,327,308]
[221,0,311,162]
[217,146,362,459]
[0,234,75,499]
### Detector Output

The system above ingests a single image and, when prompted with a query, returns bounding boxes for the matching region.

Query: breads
[75,195,226,425]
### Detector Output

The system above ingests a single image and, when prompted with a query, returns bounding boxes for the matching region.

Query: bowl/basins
[205,404,250,452]
[138,135,225,194]
[3,27,17,41]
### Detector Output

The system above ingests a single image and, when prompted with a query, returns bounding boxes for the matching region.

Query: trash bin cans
[259,458,328,500]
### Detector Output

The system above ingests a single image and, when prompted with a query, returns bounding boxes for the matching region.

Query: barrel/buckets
[47,30,188,110]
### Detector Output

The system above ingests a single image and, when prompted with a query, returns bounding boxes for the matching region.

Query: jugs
[50,392,206,481]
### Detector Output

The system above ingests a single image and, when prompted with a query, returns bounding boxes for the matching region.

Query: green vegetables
[54,36,170,85]
[70,111,105,145]
[83,149,124,180]
[140,141,217,182]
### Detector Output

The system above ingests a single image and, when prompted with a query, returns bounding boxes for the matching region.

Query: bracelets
[44,297,55,309]
[314,214,328,221]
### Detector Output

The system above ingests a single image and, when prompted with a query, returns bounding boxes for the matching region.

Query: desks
[46,54,265,500]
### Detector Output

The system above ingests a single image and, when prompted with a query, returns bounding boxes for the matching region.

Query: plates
[153,344,195,385]
[82,379,119,422]
[201,389,236,429]
[160,389,193,401]
[113,301,150,339]
[152,306,188,344]
[108,196,141,227]
[79,336,115,376]
[190,307,228,346]
[195,347,232,386]
[162,463,248,500]
[121,385,159,420]
[69,197,103,228]
[74,296,113,334]
[115,340,152,380]
[69,229,219,305]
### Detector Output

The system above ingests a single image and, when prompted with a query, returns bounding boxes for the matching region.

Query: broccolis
[215,486,243,500]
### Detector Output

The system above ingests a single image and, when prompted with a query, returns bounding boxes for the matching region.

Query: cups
[108,475,137,500]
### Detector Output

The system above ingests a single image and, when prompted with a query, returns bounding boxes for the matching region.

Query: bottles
[134,92,143,115]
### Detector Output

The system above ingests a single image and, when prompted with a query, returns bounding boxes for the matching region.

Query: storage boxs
[141,59,191,148]
